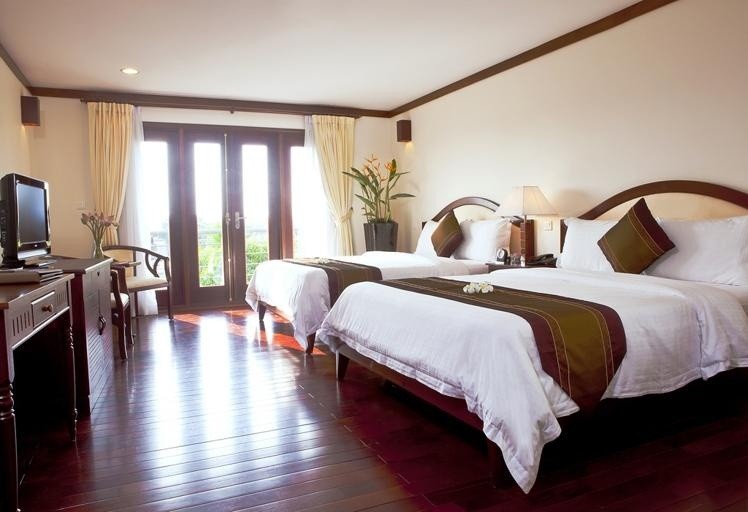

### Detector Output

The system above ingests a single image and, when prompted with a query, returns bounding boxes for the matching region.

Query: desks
[0,273,81,508]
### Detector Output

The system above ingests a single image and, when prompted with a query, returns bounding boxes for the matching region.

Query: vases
[364,223,399,253]
[90,239,106,258]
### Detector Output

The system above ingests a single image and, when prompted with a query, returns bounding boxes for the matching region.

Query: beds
[245,195,502,353]
[313,178,748,495]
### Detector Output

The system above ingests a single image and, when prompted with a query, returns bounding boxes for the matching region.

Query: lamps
[495,185,558,262]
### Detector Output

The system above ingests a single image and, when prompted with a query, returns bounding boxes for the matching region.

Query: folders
[0,269,64,285]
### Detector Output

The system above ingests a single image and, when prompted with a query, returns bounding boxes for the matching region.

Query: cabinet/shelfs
[47,257,116,416]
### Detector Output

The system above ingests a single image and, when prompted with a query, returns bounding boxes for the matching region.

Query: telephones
[527,254,557,267]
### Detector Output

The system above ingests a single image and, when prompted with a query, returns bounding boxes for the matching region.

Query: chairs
[46,254,136,362]
[97,244,173,339]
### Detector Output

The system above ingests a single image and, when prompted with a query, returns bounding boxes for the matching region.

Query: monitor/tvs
[1,173,58,266]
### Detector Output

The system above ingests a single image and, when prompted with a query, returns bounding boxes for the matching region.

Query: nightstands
[484,257,553,275]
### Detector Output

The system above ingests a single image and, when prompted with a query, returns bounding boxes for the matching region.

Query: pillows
[644,214,748,285]
[556,214,617,272]
[454,217,506,262]
[413,219,453,258]
[504,218,512,257]
[595,198,675,276]
[429,208,463,257]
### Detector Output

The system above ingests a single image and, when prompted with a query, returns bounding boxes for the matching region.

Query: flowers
[341,153,416,224]
[463,281,493,295]
[78,207,117,249]
[316,257,330,264]
